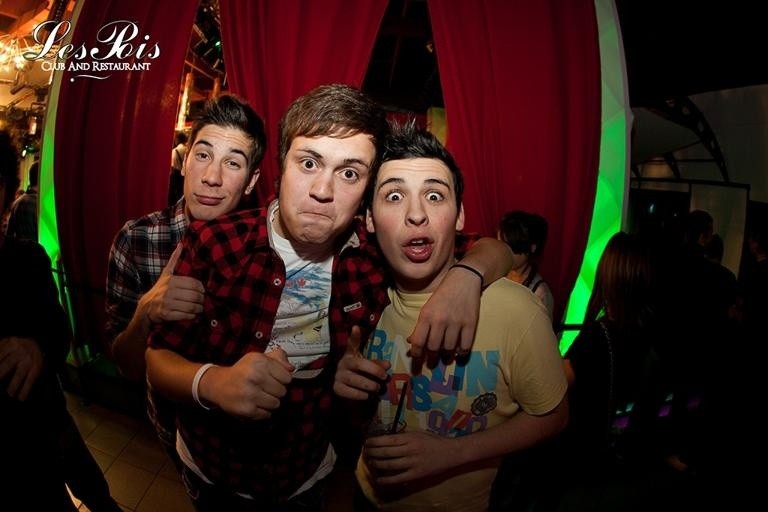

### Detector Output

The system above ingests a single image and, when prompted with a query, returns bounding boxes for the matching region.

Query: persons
[0,83,768,512]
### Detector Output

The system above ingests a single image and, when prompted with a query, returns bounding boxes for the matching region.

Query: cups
[361,417,407,479]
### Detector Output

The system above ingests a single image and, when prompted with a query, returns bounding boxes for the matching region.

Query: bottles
[18,113,40,192]
